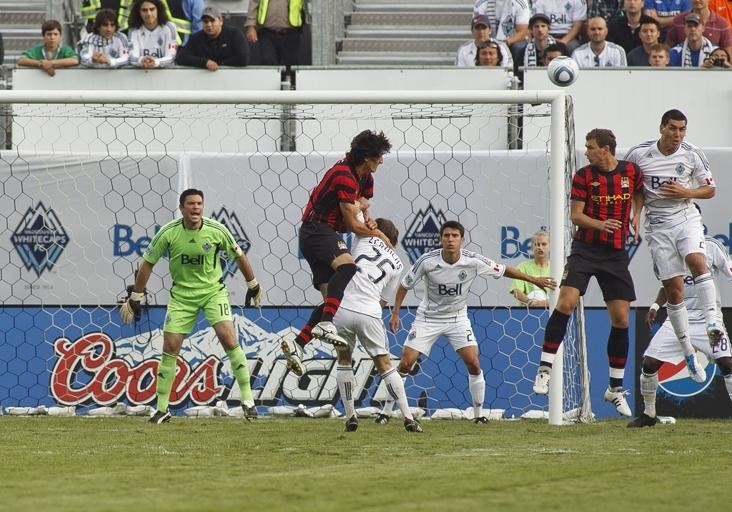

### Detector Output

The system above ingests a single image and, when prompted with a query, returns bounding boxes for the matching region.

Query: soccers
[549,54,580,87]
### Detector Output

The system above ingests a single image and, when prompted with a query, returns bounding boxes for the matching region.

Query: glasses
[478,42,498,49]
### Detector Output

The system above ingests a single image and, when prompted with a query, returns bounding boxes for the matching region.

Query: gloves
[245,278,263,309]
[119,292,144,327]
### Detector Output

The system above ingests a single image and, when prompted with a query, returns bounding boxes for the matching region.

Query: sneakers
[240,400,258,423]
[344,414,359,432]
[371,411,390,424]
[627,412,661,428]
[404,416,424,433]
[280,339,304,376]
[683,347,707,384]
[311,320,349,348]
[531,366,552,397]
[148,405,172,425]
[473,416,489,425]
[705,321,724,347]
[603,387,632,417]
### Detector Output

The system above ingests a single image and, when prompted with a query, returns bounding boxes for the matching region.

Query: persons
[626,198,732,430]
[280,128,395,376]
[117,189,262,426]
[506,225,553,309]
[453,0,732,71]
[621,107,732,399]
[533,127,646,417]
[15,0,313,71]
[372,220,558,427]
[332,217,422,437]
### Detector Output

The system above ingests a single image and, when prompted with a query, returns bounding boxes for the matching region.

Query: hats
[200,5,222,20]
[528,12,551,28]
[684,13,704,25]
[471,14,491,31]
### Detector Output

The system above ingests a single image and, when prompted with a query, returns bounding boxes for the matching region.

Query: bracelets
[648,303,661,312]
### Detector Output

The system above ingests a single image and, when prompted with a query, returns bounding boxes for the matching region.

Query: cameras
[713,58,723,66]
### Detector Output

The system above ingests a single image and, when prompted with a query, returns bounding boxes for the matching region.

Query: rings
[547,283,552,289]
